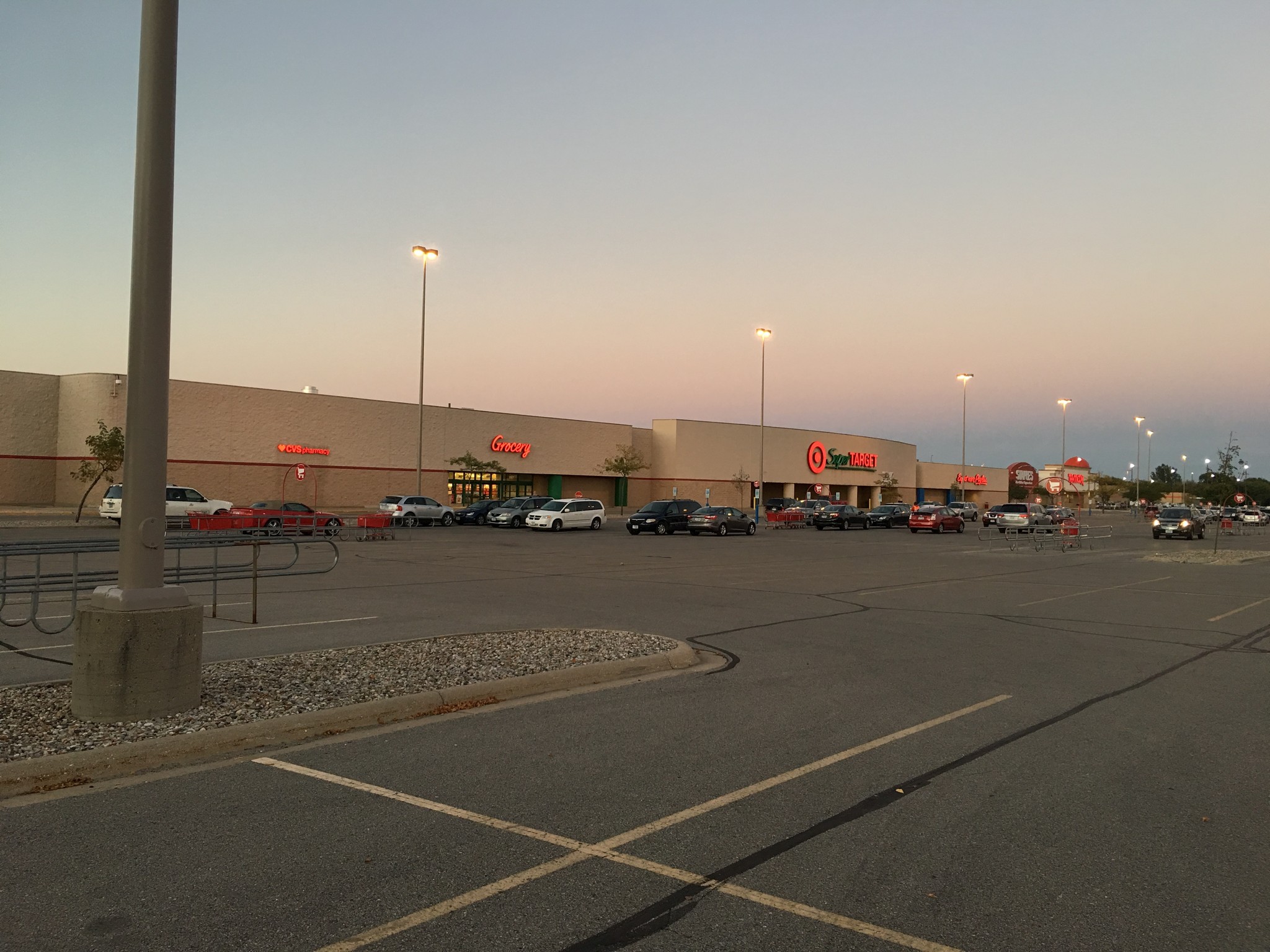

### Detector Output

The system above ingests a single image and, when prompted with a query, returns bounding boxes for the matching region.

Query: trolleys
[1060,520,1082,553]
[1218,518,1233,536]
[764,510,806,530]
[355,511,395,542]
[185,510,260,544]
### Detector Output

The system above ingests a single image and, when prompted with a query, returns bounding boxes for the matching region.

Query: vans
[997,503,1054,533]
[947,502,979,522]
[982,505,1003,527]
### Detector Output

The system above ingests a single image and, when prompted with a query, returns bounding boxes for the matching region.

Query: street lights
[1171,455,1193,503]
[412,246,438,496]
[758,328,772,507]
[1236,465,1249,505]
[1057,399,1072,506]
[957,374,974,500]
[1205,459,1215,486]
[1127,417,1153,505]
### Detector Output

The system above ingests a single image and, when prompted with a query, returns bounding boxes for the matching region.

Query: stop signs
[575,492,583,498]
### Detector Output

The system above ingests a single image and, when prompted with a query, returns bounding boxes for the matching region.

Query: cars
[1042,505,1075,530]
[687,506,756,536]
[1095,501,1130,509]
[784,495,914,530]
[455,495,607,532]
[909,501,965,534]
[229,500,345,535]
[1144,503,1270,526]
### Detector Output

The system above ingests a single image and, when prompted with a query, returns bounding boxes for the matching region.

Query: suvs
[1152,507,1206,540]
[378,495,455,526]
[99,482,234,525]
[765,497,799,511]
[626,499,702,535]
